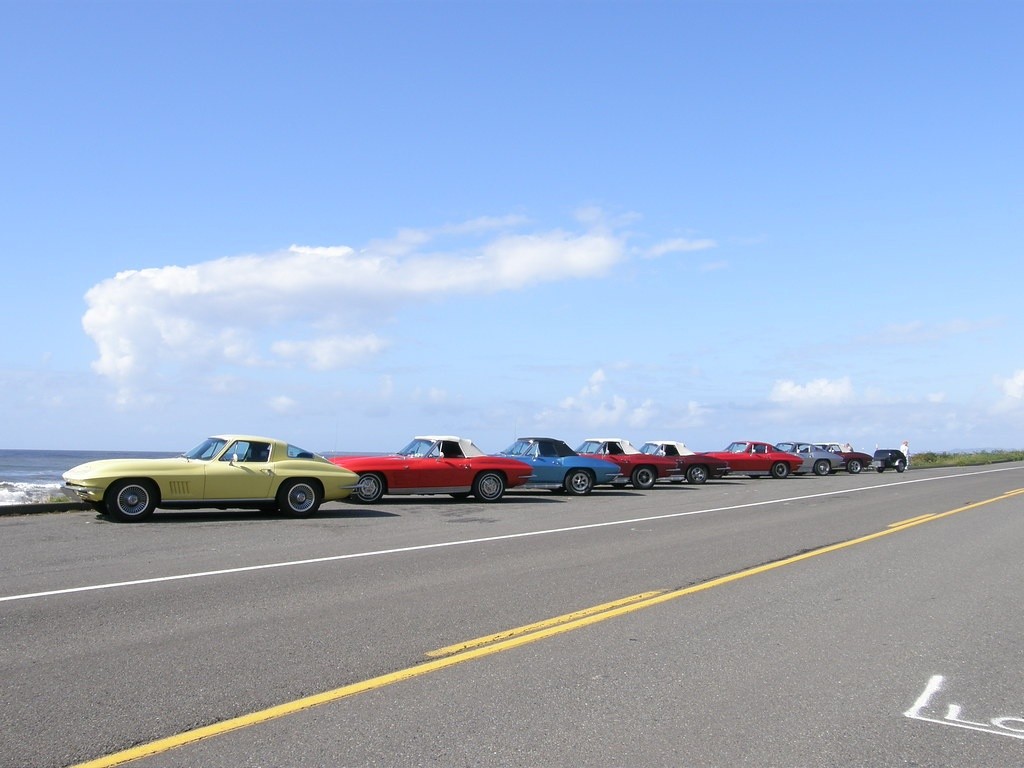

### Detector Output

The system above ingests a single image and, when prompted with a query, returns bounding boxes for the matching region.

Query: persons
[846,443,854,453]
[899,440,911,471]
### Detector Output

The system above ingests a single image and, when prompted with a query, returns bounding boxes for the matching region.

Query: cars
[863,449,907,473]
[719,441,803,481]
[775,441,843,476]
[813,442,873,474]
[639,440,730,484]
[485,437,622,495]
[573,438,677,489]
[59,433,360,525]
[317,434,533,503]
